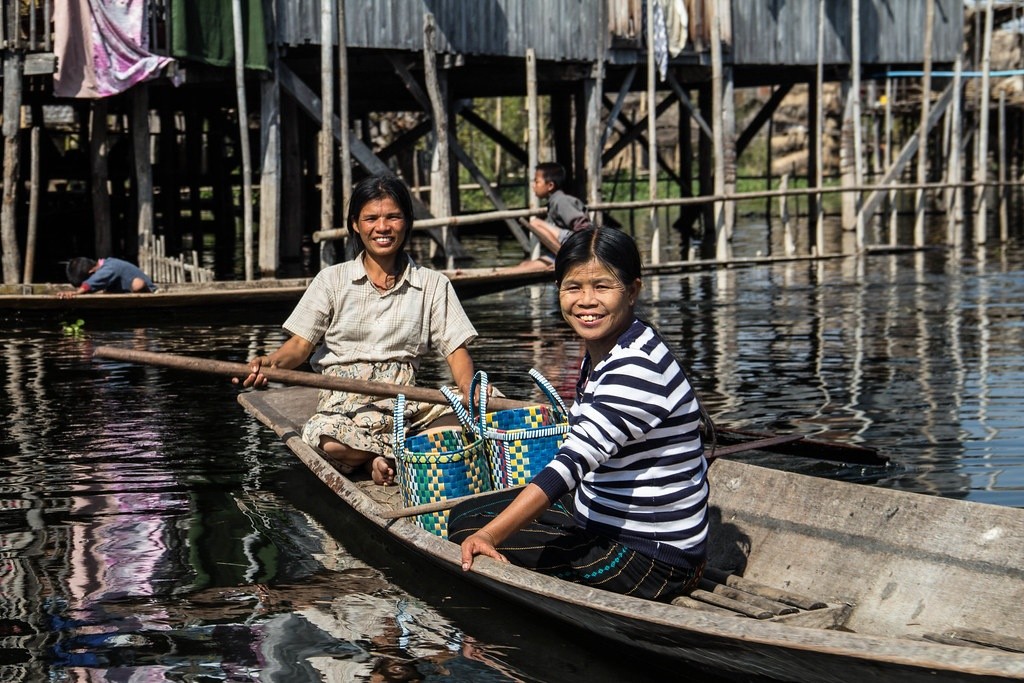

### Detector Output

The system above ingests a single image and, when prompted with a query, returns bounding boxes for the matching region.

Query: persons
[519,161,594,269]
[448,225,718,603]
[231,173,508,488]
[57,256,159,300]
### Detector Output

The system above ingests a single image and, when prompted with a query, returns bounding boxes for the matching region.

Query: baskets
[392,385,493,542]
[467,368,570,491]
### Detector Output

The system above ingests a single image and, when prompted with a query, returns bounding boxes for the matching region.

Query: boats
[0,267,559,323]
[238,382,1023,683]
